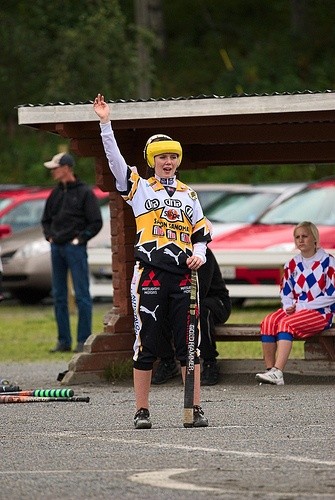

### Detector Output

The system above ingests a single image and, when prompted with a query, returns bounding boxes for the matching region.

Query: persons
[92,92,212,428]
[39,152,103,355]
[254,220,335,385]
[150,237,232,386]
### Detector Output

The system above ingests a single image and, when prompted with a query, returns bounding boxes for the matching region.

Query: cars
[203,181,306,242]
[209,179,335,309]
[0,184,240,303]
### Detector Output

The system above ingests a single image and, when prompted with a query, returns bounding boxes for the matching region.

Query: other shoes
[74,343,84,352]
[49,345,69,352]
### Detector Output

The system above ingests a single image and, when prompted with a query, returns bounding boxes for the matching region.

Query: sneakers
[150,361,180,384]
[134,408,152,428]
[202,360,219,386]
[255,367,284,385]
[184,406,208,426]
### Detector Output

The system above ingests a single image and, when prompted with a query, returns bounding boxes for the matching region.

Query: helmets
[145,134,184,168]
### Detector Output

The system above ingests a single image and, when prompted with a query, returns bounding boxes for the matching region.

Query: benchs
[214,324,335,364]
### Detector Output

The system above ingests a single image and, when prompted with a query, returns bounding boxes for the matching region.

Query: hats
[44,152,72,168]
